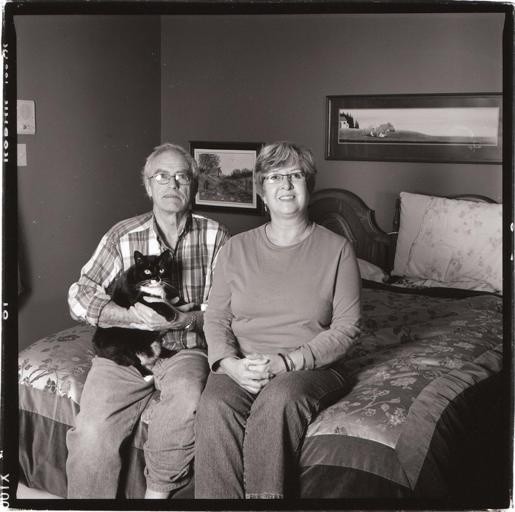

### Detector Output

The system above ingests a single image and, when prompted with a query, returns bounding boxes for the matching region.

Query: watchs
[183,310,197,331]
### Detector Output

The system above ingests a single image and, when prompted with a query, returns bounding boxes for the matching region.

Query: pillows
[356,252,389,285]
[392,190,505,299]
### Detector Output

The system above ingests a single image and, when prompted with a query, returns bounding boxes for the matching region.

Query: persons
[64,141,233,499]
[194,140,364,498]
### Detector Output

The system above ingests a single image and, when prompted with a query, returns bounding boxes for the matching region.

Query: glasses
[147,171,193,185]
[262,173,303,184]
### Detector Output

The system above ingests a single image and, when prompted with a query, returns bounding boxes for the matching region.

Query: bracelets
[277,352,291,372]
[283,352,294,372]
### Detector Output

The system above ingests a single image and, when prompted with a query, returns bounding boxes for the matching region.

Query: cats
[93,253,209,383]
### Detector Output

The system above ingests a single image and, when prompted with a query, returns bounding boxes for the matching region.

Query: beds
[17,188,502,500]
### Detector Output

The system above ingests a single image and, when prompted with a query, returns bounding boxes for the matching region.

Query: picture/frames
[324,92,504,163]
[185,136,267,216]
